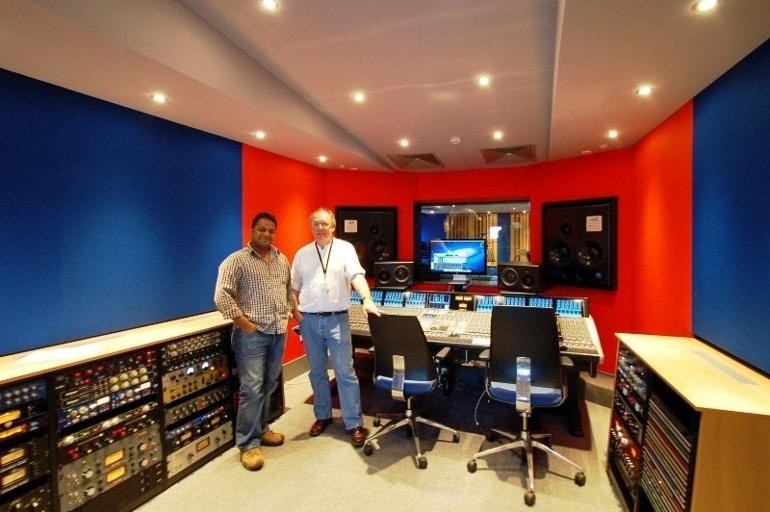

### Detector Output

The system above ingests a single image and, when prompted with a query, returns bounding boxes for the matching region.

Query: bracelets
[362,297,372,300]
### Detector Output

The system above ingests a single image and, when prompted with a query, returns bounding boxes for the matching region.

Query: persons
[291,206,386,446]
[214,209,292,471]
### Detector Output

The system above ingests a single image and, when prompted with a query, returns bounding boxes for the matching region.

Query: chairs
[346,311,460,469]
[466,306,586,507]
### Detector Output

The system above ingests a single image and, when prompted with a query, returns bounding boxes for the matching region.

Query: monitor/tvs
[429,238,487,285]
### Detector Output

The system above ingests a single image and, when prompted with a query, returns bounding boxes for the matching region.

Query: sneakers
[240,448,264,471]
[260,430,284,445]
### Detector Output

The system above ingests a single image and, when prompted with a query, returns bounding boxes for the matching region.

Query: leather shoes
[351,427,365,446]
[310,417,332,436]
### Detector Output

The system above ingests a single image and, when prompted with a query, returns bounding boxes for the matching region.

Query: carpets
[304,354,591,451]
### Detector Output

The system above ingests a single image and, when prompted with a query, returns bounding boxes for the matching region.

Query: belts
[311,310,348,316]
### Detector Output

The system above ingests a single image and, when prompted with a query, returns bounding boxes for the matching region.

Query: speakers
[374,262,414,287]
[541,196,618,290]
[498,263,544,293]
[336,207,397,277]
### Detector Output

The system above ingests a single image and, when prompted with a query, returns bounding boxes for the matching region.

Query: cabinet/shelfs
[606,333,770,512]
[0,323,285,512]
